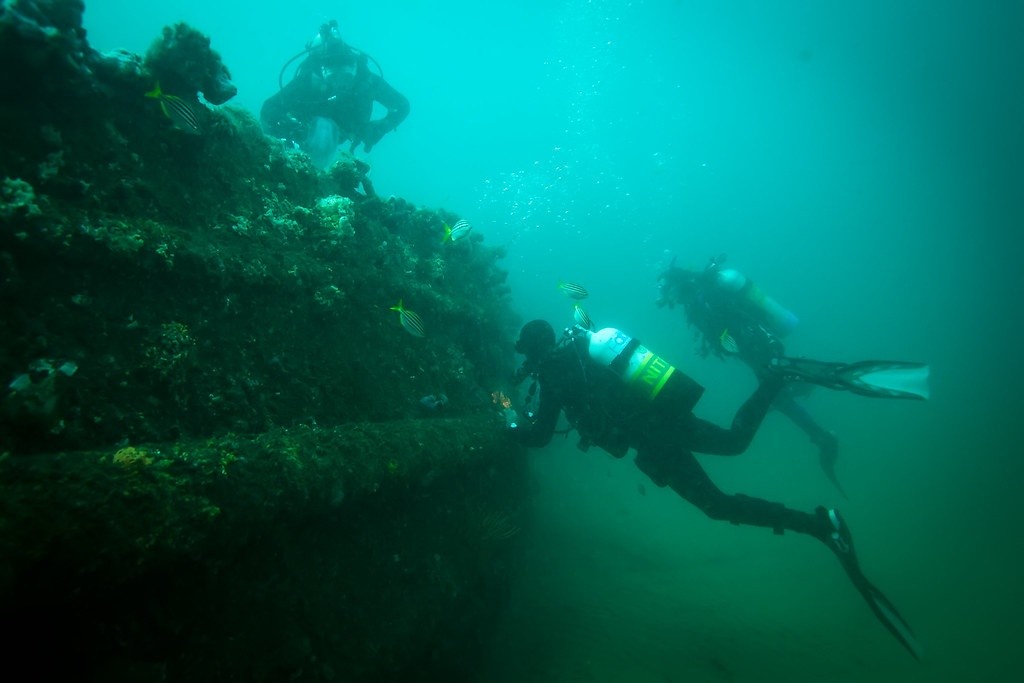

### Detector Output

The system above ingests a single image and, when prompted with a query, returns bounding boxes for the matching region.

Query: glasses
[656,284,669,291]
[320,62,358,82]
[514,340,531,354]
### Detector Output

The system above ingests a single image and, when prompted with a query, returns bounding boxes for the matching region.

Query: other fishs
[563,281,596,331]
[388,297,428,342]
[717,327,740,354]
[142,77,209,141]
[440,219,472,245]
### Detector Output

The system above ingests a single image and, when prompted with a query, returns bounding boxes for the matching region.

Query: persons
[659,268,839,464]
[260,45,410,153]
[502,321,860,569]
